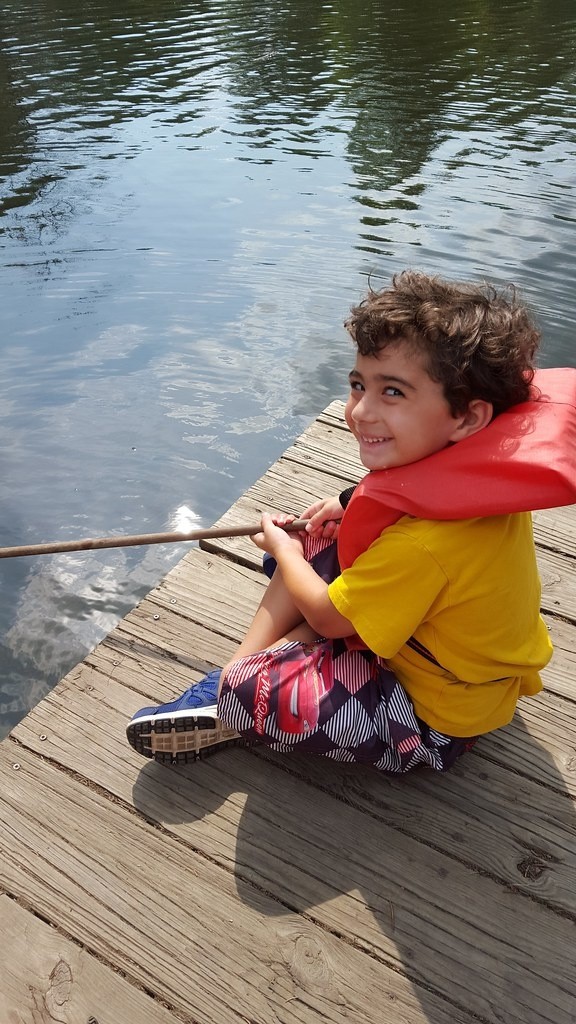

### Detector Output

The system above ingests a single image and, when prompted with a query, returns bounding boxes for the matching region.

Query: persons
[125,269,555,781]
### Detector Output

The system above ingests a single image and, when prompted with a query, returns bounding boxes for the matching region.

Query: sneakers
[126,669,261,765]
[263,553,276,577]
